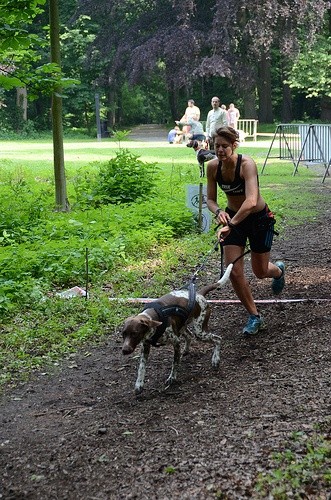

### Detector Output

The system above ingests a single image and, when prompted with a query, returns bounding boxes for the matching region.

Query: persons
[175,114,206,146]
[207,126,285,334]
[206,96,228,150]
[220,103,240,130]
[168,126,185,144]
[180,99,200,136]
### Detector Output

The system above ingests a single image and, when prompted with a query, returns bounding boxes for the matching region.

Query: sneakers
[243,314,265,335]
[271,261,285,294]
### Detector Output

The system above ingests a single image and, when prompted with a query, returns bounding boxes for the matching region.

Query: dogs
[120,249,252,395]
[186,140,217,178]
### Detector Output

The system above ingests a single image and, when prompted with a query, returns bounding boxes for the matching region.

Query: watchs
[215,208,222,217]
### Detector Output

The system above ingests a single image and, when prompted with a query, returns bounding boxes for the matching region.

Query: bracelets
[227,221,235,229]
